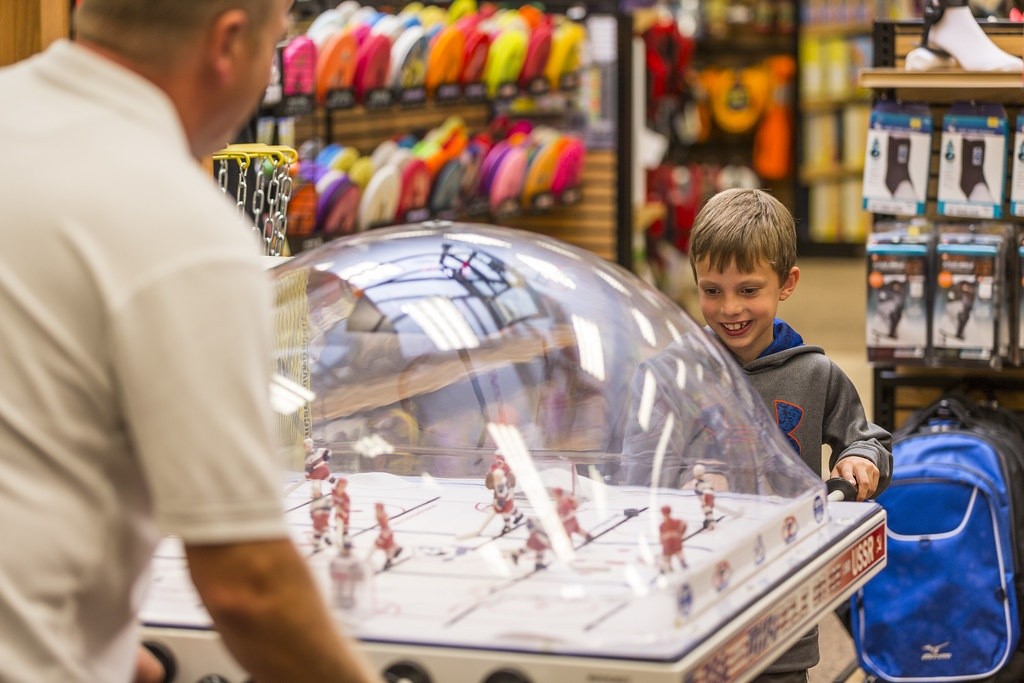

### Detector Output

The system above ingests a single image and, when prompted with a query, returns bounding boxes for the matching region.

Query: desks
[128,469,887,683]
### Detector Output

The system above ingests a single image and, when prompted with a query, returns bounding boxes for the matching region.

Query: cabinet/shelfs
[210,0,912,467]
[826,20,1024,683]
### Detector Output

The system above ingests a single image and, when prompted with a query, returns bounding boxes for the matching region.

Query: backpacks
[850,396,1024,683]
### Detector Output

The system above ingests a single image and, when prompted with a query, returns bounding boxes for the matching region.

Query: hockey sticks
[474,502,492,512]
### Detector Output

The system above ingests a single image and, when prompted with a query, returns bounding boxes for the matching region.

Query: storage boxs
[861,102,934,217]
[1008,110,1024,218]
[937,102,1008,218]
[864,222,1024,369]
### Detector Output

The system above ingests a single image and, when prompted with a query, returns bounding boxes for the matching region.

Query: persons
[692,465,716,529]
[0,0,386,683]
[621,187,894,683]
[485,449,591,569]
[659,506,688,571]
[303,438,402,610]
[348,240,543,483]
